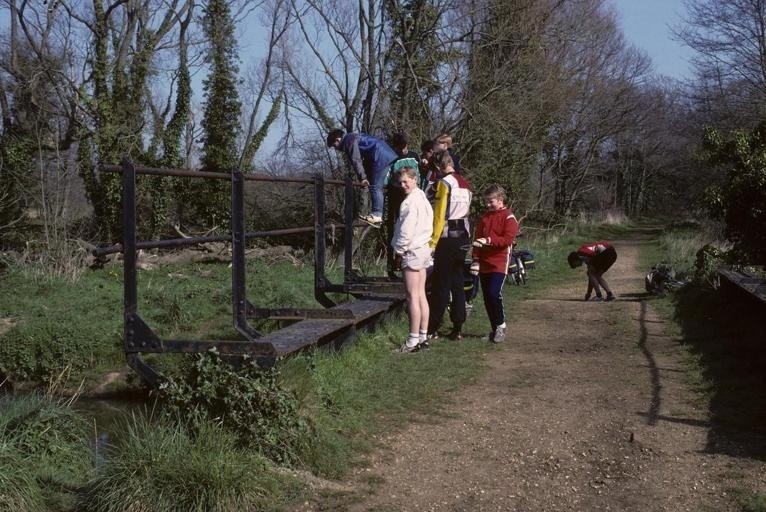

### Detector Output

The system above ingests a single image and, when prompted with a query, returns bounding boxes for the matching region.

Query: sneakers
[482,325,509,344]
[446,329,464,340]
[427,330,439,339]
[590,294,616,302]
[392,340,431,356]
[358,213,382,228]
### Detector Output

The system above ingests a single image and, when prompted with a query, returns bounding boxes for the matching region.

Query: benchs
[716,262,766,311]
[239,278,426,390]
[741,279,766,318]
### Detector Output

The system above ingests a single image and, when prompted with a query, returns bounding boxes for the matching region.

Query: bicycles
[645,261,689,296]
[508,233,536,286]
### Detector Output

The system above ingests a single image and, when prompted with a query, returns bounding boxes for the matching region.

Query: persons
[567,242,617,302]
[434,133,463,176]
[421,141,440,207]
[390,165,435,356]
[327,129,399,229]
[419,159,429,190]
[387,134,420,283]
[427,150,473,341]
[468,184,520,344]
